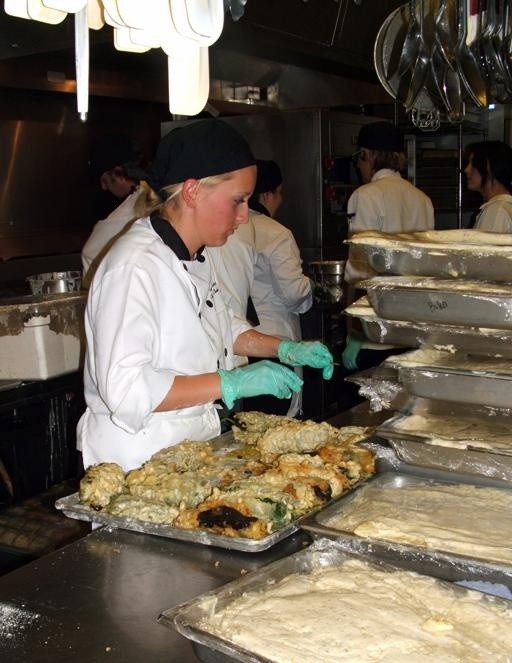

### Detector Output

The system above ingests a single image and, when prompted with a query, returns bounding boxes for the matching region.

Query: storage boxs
[0,293,88,381]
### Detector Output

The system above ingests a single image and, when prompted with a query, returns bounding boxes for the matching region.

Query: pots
[373,0,464,131]
[26,270,84,295]
[309,260,346,302]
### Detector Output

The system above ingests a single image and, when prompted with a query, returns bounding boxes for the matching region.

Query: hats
[257,159,283,192]
[357,120,405,153]
[91,142,130,181]
[128,119,256,193]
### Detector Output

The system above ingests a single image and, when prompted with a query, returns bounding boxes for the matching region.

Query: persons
[463,136,512,234]
[204,214,250,372]
[76,112,338,478]
[341,119,437,405]
[230,155,315,420]
[83,135,160,281]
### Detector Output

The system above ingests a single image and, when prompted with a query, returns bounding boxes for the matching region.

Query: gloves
[217,360,304,410]
[278,338,334,380]
[341,329,362,370]
